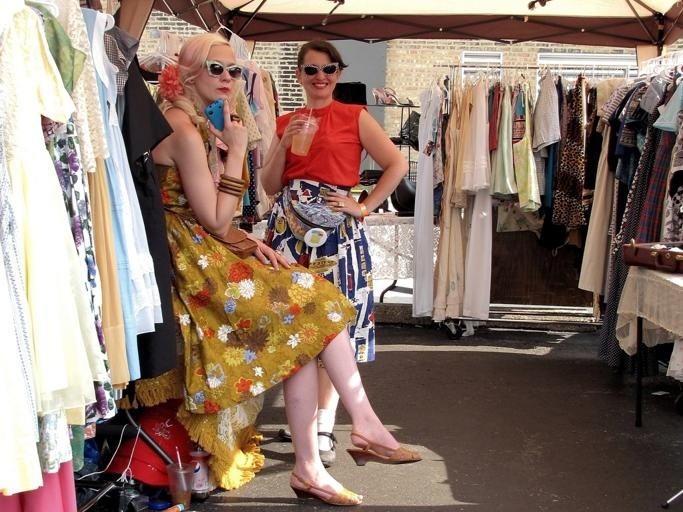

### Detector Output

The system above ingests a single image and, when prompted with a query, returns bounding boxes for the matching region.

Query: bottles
[189,448,209,494]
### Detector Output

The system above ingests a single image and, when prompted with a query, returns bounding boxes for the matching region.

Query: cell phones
[205,99,223,132]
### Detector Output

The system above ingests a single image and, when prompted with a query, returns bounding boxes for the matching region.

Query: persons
[259,38,408,467]
[150,34,424,506]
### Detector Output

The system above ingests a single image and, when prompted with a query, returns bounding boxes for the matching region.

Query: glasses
[202,59,244,81]
[297,62,340,76]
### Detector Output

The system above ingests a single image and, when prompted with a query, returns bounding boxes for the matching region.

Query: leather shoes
[315,430,337,468]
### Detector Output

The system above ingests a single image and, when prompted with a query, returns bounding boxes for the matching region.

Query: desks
[617,265,683,427]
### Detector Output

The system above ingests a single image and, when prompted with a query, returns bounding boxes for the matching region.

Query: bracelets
[213,172,247,197]
[356,202,370,215]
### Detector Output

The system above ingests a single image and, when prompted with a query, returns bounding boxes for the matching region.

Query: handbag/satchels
[281,184,350,247]
[399,110,422,153]
[202,220,259,262]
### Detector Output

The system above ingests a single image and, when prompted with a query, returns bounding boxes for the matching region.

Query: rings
[229,111,242,123]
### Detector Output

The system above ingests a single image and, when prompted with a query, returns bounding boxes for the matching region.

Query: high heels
[289,471,365,507]
[345,433,423,471]
[373,85,413,107]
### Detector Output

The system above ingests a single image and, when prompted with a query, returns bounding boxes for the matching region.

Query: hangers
[29,1,115,32]
[428,49,683,89]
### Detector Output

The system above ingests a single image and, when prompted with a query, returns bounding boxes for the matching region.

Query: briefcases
[621,238,683,274]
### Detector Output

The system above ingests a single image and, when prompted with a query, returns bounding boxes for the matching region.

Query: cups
[167,464,194,511]
[291,112,317,155]
